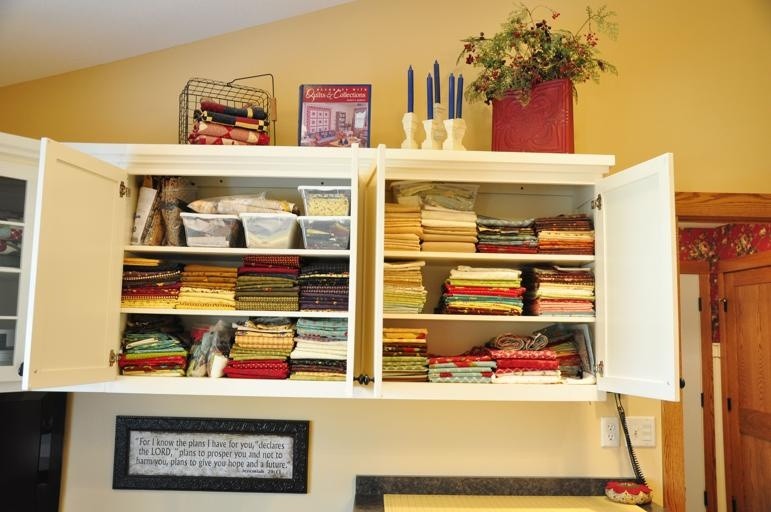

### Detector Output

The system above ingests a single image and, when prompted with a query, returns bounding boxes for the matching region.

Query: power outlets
[600,416,620,449]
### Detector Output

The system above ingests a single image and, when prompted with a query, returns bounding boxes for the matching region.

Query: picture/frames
[112,414,310,496]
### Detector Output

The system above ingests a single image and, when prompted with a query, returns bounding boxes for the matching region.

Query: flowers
[455,2,620,108]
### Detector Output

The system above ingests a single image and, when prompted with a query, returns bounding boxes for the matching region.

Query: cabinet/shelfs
[0,130,682,411]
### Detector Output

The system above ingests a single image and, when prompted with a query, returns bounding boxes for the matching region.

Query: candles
[407,60,463,120]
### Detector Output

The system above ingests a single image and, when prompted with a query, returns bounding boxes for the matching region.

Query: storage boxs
[178,179,351,251]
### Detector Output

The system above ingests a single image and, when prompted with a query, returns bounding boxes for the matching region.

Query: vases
[490,78,575,153]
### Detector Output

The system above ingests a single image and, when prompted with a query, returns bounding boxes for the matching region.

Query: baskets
[178,73,278,146]
